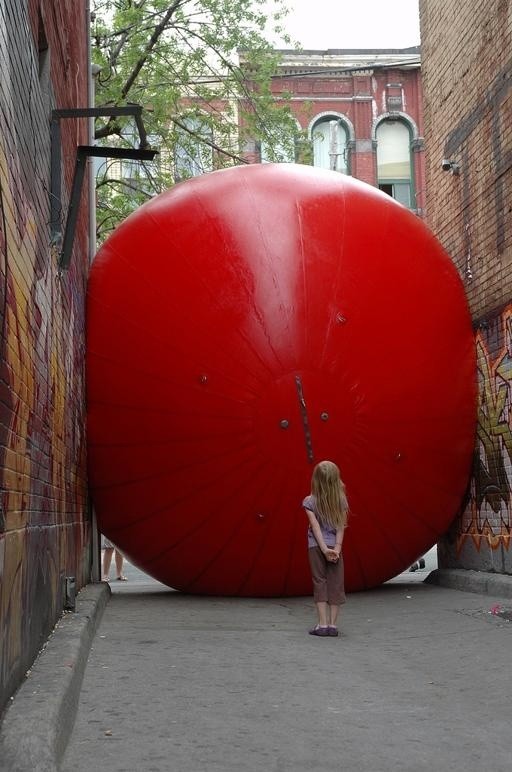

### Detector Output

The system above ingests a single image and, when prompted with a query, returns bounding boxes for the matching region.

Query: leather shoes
[309,625,338,636]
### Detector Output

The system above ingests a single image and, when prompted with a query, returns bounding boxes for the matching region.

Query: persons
[301,460,349,636]
[101,534,129,582]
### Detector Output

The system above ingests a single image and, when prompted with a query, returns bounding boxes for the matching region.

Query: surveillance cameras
[441,160,450,172]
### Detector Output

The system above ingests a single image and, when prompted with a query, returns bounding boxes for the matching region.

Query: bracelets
[334,543,342,545]
